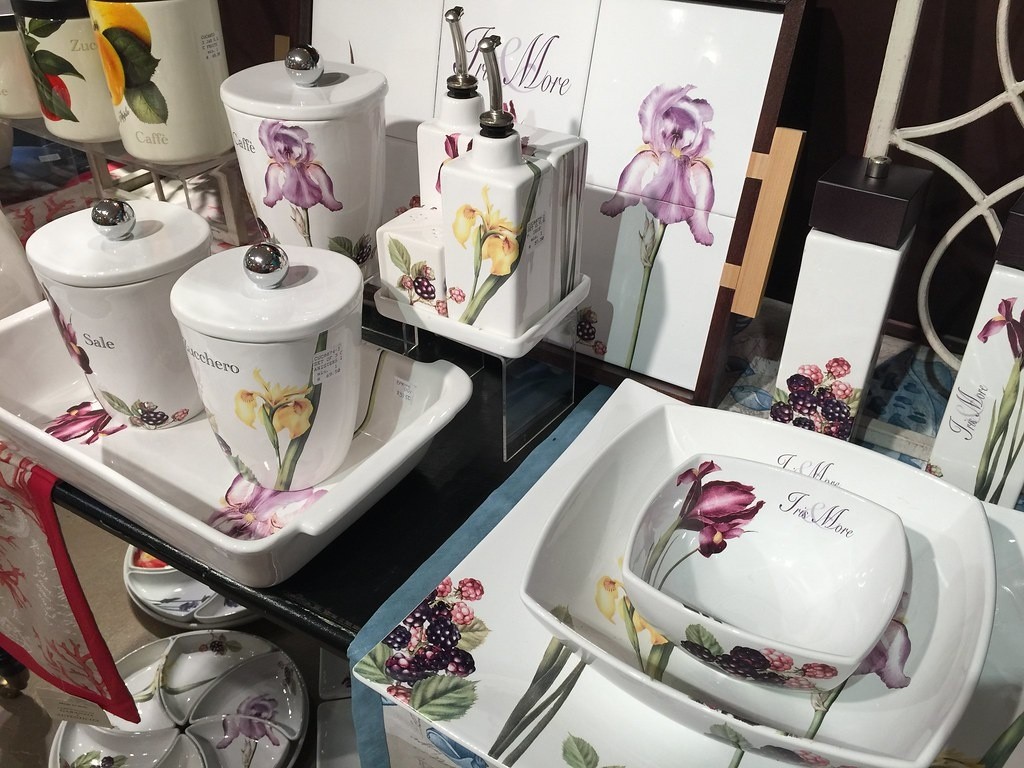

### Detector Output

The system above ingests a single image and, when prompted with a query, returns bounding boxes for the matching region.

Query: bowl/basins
[619,451,909,702]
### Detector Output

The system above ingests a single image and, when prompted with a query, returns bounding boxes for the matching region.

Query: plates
[122,541,266,627]
[1,291,473,588]
[519,400,997,767]
[46,628,314,768]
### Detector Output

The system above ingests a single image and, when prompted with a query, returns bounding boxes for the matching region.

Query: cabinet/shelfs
[1,117,249,249]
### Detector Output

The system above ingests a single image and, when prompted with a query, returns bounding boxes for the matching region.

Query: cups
[172,241,364,494]
[8,1,122,146]
[0,11,43,121]
[221,45,394,275]
[23,199,212,428]
[85,0,238,170]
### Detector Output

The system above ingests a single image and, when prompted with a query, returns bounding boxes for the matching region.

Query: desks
[0,295,619,662]
